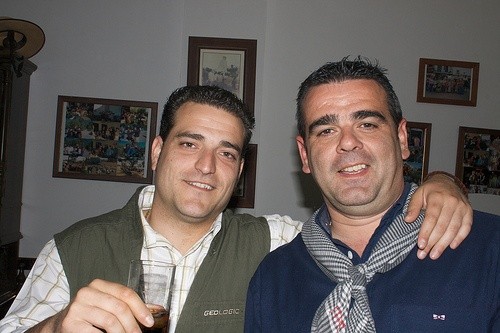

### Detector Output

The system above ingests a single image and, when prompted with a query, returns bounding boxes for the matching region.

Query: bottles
[13,263,26,297]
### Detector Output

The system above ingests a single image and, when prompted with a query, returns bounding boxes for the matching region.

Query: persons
[201,54,240,98]
[59,101,151,179]
[463,132,500,194]
[243,59,485,331]
[424,63,470,100]
[403,126,426,185]
[0,84,473,333]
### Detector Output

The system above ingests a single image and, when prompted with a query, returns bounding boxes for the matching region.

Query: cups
[125,260,176,333]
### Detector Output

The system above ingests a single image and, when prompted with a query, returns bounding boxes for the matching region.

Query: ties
[301,182,424,333]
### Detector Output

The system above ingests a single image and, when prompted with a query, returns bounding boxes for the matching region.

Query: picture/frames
[52,95,158,184]
[187,36,257,127]
[398,121,432,184]
[222,143,257,208]
[417,58,480,107]
[455,126,500,195]
